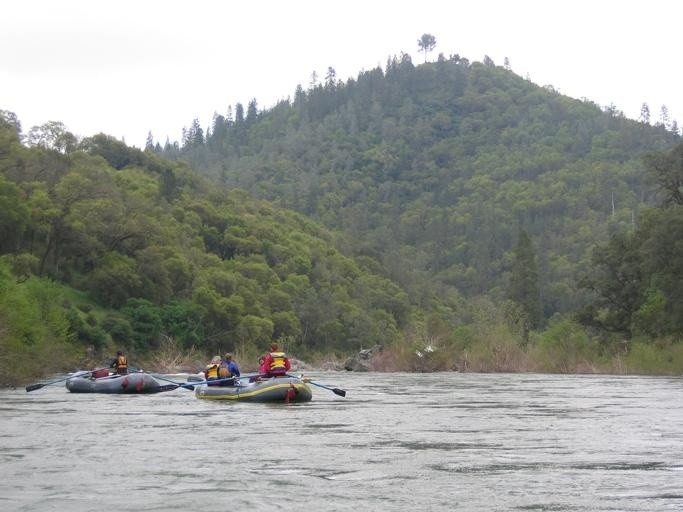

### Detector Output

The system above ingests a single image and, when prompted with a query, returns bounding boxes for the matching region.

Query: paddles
[285,374,345,397]
[26,365,115,393]
[156,374,263,391]
[128,368,194,391]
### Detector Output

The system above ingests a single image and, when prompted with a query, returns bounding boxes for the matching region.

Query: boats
[194,378,311,403]
[66,367,159,394]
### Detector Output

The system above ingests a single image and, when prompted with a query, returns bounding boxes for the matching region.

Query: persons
[250,357,266,382]
[218,353,240,387]
[205,356,222,386]
[265,343,291,378]
[110,351,127,375]
